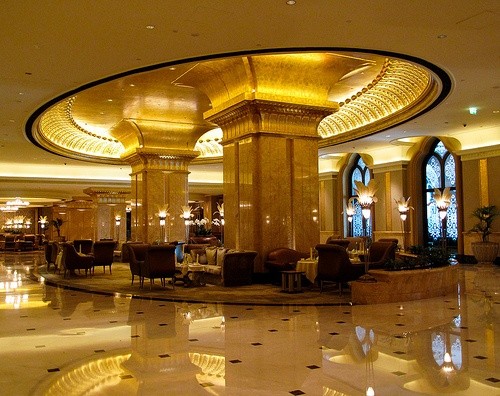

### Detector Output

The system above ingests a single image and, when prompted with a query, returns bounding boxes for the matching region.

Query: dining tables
[55,250,91,275]
[298,257,362,285]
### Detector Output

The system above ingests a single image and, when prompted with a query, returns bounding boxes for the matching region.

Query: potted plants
[51,218,66,241]
[470,204,500,264]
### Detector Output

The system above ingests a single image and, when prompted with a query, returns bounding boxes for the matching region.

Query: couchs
[190,246,258,286]
[265,247,317,286]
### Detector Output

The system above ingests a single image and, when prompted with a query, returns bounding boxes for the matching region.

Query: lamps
[180,205,195,225]
[433,187,453,220]
[114,215,121,226]
[394,196,415,222]
[7,197,30,207]
[37,215,49,229]
[1,215,33,229]
[212,202,225,226]
[154,208,171,227]
[346,179,381,227]
[0,206,19,213]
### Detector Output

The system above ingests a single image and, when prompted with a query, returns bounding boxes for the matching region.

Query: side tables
[280,270,305,293]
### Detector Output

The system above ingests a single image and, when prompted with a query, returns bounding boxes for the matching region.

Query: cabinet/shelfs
[461,232,500,258]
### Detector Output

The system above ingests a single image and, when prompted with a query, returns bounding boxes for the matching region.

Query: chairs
[127,243,154,288]
[183,244,212,253]
[4,235,16,251]
[93,240,115,275]
[24,236,35,250]
[189,236,218,245]
[142,244,176,291]
[362,241,394,267]
[315,243,366,296]
[63,242,93,277]
[46,239,60,272]
